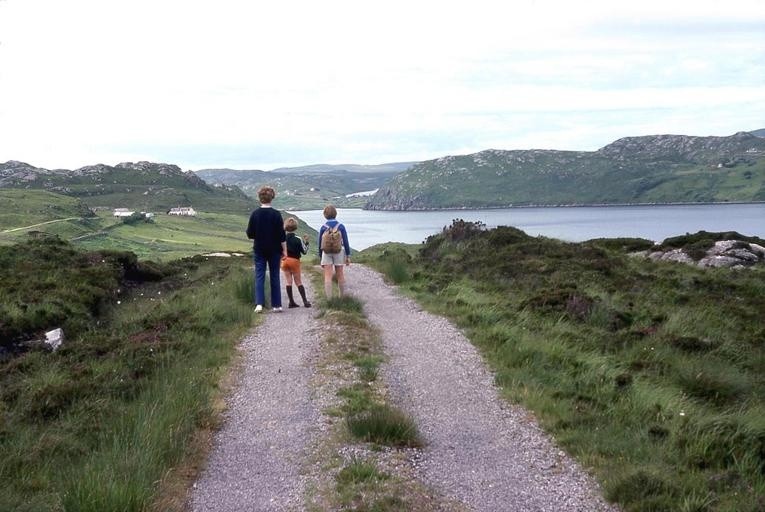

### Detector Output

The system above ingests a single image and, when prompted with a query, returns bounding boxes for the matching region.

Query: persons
[280,217,311,308]
[318,204,351,302]
[246,185,288,313]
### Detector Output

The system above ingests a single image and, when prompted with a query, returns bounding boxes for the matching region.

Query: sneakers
[272,307,282,312]
[304,302,311,307]
[253,304,263,314]
[289,302,299,308]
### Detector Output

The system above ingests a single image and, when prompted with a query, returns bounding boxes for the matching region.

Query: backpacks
[320,222,342,254]
